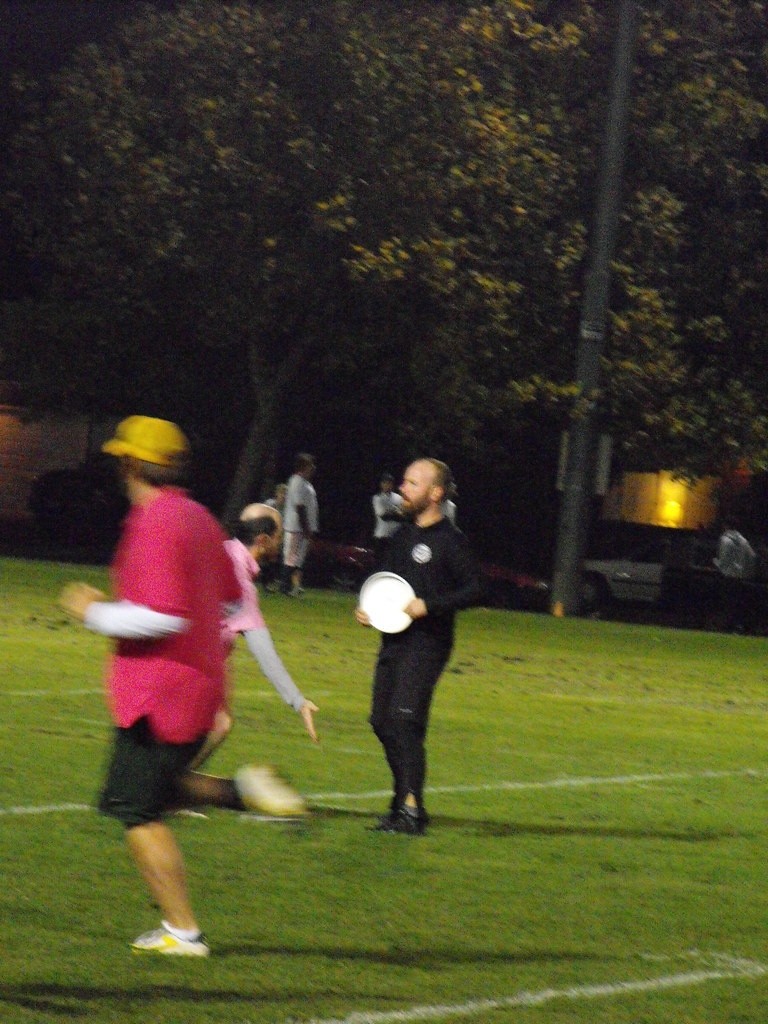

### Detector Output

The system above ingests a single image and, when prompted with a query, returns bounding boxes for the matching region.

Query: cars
[570,534,766,623]
[306,516,552,608]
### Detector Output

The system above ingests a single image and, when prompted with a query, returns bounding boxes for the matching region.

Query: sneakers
[369,804,427,837]
[130,916,210,959]
[233,762,307,817]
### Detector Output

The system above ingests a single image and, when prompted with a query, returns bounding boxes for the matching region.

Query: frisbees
[358,571,416,634]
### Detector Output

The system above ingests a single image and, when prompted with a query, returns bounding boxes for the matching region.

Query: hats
[100,415,191,468]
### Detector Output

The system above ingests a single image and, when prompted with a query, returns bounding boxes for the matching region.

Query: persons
[440,475,460,531]
[176,503,319,820]
[355,458,477,836]
[58,415,306,953]
[372,473,405,561]
[710,516,754,634]
[264,453,318,596]
